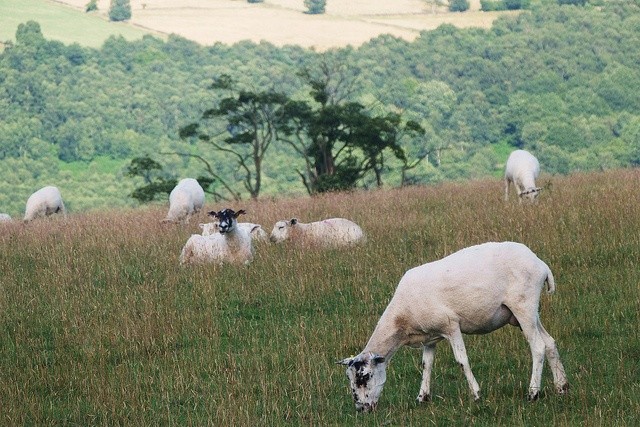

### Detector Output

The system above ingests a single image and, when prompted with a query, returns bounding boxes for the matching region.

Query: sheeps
[268,217,366,254]
[332,241,571,415]
[503,148,544,209]
[177,208,256,269]
[198,222,270,246]
[160,177,206,225]
[21,185,66,226]
